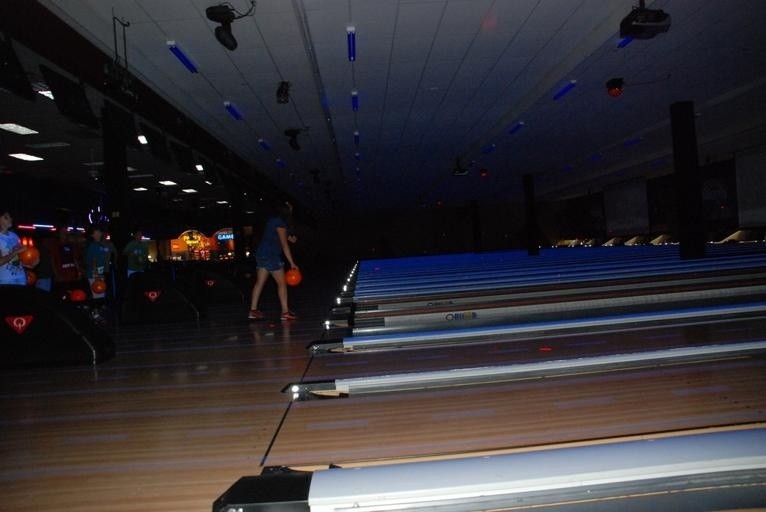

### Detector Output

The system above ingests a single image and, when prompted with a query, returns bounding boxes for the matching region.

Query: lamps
[277,81,291,103]
[223,101,244,121]
[205,5,238,51]
[352,90,359,111]
[347,25,357,62]
[285,131,300,152]
[166,41,198,75]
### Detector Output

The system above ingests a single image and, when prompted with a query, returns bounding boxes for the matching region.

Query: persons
[39,214,91,297]
[246,201,302,322]
[0,207,42,288]
[79,223,118,325]
[118,228,152,281]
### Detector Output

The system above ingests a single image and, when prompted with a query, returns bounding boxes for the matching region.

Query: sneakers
[246,308,264,318]
[278,312,295,321]
